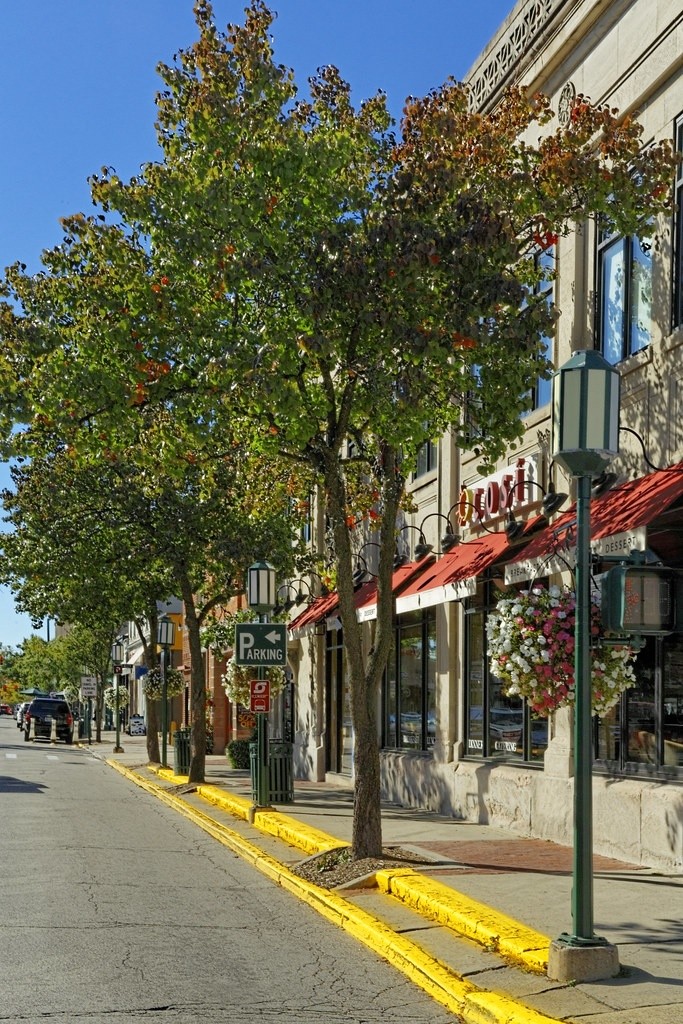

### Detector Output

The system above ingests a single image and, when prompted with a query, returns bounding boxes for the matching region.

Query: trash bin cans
[249,737,294,806]
[171,725,193,777]
[78,716,86,738]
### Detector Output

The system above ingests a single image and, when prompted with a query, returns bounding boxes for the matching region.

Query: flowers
[221,656,287,712]
[484,584,642,718]
[141,667,186,702]
[103,686,129,715]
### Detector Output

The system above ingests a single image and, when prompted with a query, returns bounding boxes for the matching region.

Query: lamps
[503,480,579,545]
[274,542,383,617]
[414,513,483,563]
[111,634,128,646]
[589,427,682,502]
[440,501,506,555]
[540,460,570,519]
[392,526,457,574]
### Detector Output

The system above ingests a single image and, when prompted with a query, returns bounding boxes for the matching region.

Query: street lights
[548,348,623,980]
[245,559,277,809]
[156,613,176,769]
[111,639,125,754]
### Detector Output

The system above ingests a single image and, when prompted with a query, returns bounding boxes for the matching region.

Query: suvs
[0,704,13,715]
[13,698,75,745]
